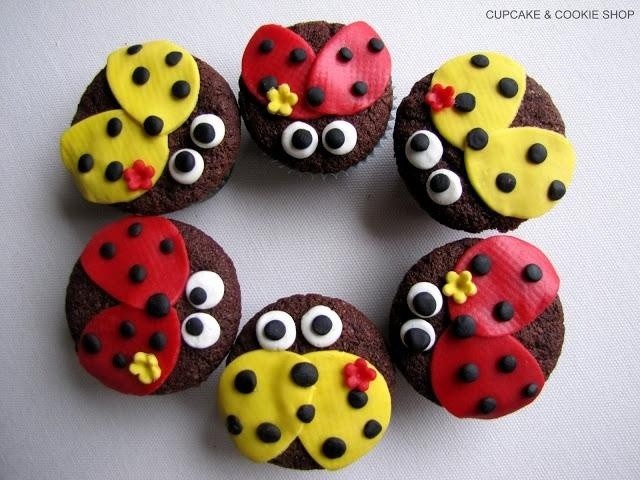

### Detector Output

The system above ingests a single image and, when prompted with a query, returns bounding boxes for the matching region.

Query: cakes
[57,16,577,473]
